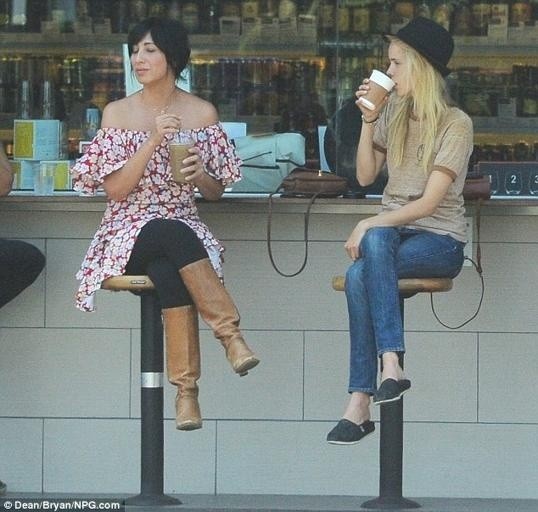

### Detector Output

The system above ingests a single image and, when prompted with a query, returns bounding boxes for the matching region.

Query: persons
[324,15,476,448]
[72,12,259,431]
[0,147,46,312]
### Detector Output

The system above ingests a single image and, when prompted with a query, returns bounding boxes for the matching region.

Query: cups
[168,143,193,182]
[356,68,396,111]
[33,164,56,197]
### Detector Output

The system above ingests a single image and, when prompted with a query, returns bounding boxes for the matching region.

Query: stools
[100,275,183,506]
[332,274,454,510]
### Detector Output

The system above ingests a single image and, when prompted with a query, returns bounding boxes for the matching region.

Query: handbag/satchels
[227,132,307,194]
[278,167,349,198]
[464,170,494,200]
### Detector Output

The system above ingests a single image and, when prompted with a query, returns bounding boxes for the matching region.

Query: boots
[179,257,260,375]
[161,304,204,430]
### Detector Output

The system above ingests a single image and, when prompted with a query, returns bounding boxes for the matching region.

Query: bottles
[444,65,538,198]
[1,0,538,36]
[281,103,327,163]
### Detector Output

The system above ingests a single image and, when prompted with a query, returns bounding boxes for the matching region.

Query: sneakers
[373,380,412,404]
[327,419,375,444]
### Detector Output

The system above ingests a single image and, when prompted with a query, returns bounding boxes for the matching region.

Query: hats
[386,16,455,76]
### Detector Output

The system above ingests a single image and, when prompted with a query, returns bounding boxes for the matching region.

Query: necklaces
[140,90,177,117]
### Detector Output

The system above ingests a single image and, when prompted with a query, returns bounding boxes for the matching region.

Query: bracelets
[361,113,381,127]
